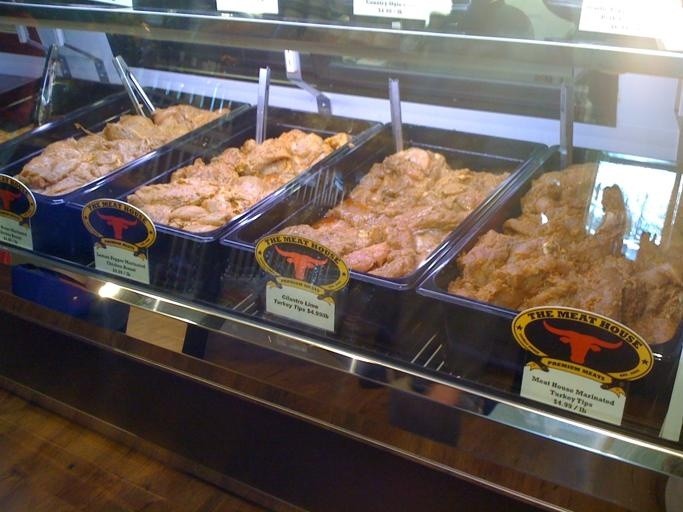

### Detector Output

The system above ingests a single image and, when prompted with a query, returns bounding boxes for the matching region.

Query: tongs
[113,54,156,116]
[27,42,58,131]
